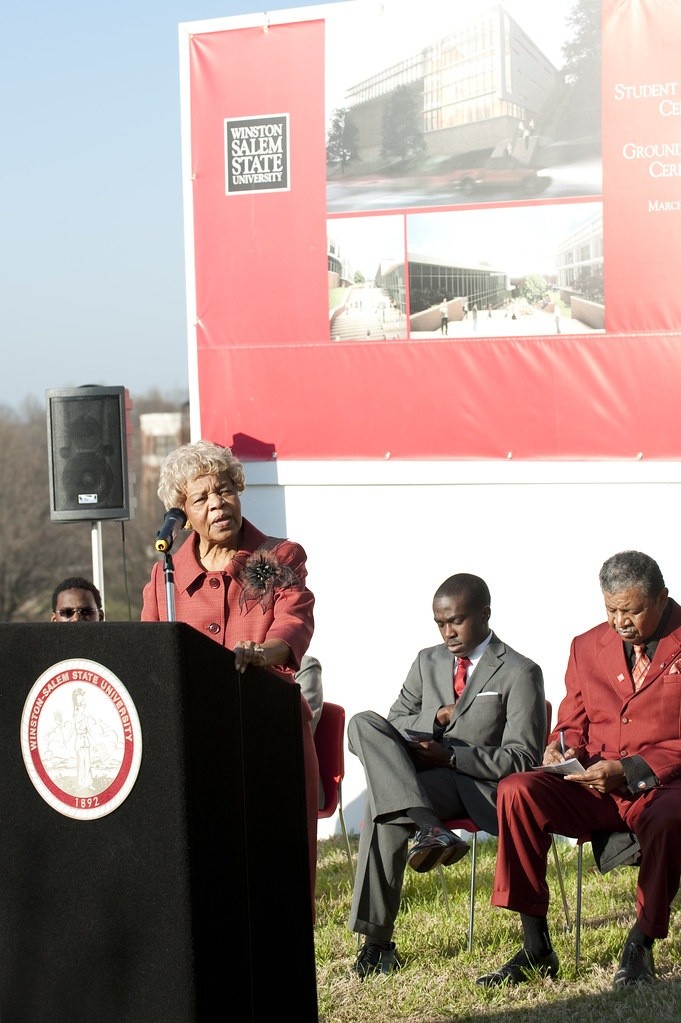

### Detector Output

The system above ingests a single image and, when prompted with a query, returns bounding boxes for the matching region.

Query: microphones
[155,507,188,552]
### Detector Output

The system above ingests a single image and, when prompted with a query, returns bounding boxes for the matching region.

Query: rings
[256,649,264,654]
[589,785,594,789]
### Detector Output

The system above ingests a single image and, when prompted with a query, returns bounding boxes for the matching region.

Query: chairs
[437,701,570,953]
[573,833,641,975]
[314,702,355,891]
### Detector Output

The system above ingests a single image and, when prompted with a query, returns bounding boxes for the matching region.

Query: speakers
[46,384,130,523]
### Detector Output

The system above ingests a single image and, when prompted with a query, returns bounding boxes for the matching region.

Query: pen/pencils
[559,730,565,755]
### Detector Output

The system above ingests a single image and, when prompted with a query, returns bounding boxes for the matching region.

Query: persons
[51,577,104,622]
[440,295,560,335]
[506,119,535,155]
[347,574,548,980]
[141,441,318,924]
[479,552,681,987]
[293,656,325,810]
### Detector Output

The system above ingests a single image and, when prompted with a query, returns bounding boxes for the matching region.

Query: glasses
[54,607,100,619]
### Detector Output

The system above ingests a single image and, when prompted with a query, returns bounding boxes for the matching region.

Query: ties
[453,656,473,704]
[630,643,651,693]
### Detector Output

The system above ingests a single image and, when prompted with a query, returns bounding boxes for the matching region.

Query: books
[532,757,586,776]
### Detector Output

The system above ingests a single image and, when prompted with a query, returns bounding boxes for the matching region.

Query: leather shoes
[613,941,656,984]
[353,942,400,982]
[406,823,471,873]
[476,948,559,988]
